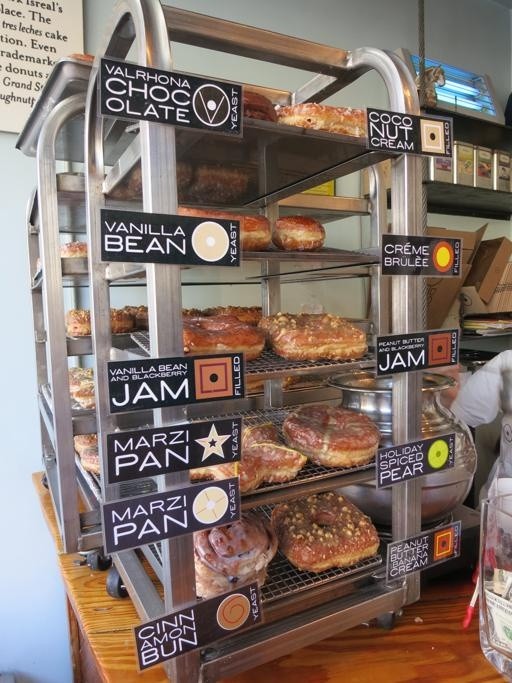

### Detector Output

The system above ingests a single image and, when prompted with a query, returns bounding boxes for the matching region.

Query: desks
[60,557,512,683]
[35,469,86,557]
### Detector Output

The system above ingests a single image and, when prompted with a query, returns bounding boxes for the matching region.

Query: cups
[478,493,512,682]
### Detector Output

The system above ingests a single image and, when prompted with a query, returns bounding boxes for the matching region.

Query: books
[463,318,512,337]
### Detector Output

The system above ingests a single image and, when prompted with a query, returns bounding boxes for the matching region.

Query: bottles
[326,372,479,542]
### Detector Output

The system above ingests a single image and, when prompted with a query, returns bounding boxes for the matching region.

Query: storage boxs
[425,224,511,331]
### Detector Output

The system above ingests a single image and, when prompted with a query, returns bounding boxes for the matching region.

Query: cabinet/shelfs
[414,106,512,504]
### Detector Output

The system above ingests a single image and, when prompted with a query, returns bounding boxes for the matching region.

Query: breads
[73,406,382,492]
[191,492,381,599]
[69,366,328,410]
[64,306,369,363]
[60,53,367,138]
[60,206,326,259]
